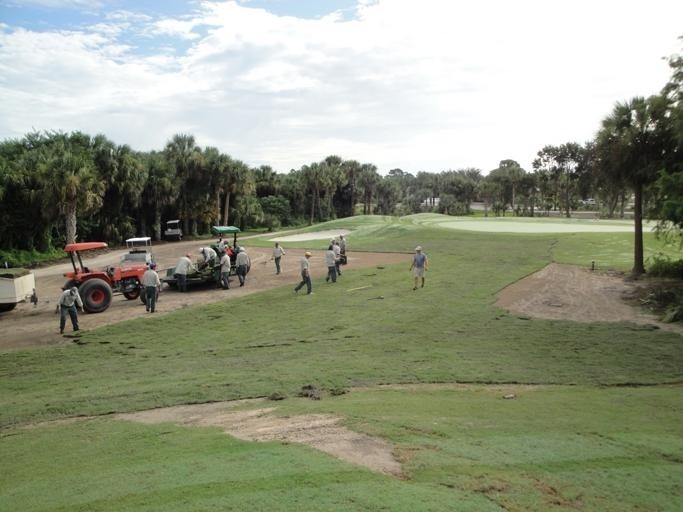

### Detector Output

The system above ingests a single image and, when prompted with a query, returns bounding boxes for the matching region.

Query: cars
[582,198,595,204]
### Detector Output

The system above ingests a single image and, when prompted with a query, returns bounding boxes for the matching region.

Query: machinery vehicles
[212,226,251,278]
[59,238,160,314]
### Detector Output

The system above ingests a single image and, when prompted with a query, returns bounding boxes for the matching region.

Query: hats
[305,252,312,257]
[199,248,203,253]
[150,263,156,267]
[71,287,78,296]
[239,246,245,251]
[415,246,422,251]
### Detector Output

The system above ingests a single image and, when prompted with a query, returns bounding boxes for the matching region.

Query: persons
[141,263,161,312]
[271,242,285,275]
[198,240,249,290]
[408,245,428,291]
[294,251,314,294]
[174,253,196,291]
[54,286,84,334]
[325,233,346,282]
